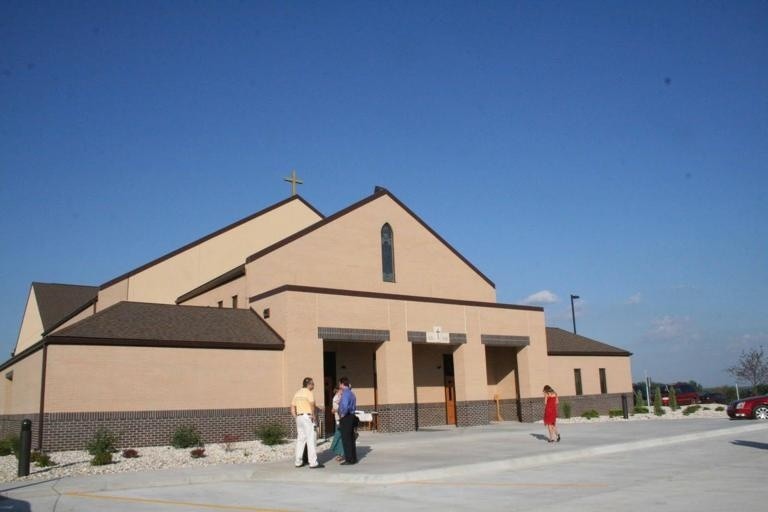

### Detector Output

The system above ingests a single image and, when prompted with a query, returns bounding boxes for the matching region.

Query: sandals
[549,434,561,442]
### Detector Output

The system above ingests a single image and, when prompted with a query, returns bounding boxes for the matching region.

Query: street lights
[571,294,579,334]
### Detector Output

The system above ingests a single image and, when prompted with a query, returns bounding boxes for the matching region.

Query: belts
[297,413,311,415]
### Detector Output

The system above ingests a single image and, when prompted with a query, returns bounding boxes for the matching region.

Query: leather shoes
[337,455,354,465]
[311,465,325,468]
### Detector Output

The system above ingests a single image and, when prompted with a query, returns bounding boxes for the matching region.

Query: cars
[642,383,768,420]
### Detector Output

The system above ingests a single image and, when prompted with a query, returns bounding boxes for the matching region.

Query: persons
[291,377,357,467]
[543,385,560,443]
